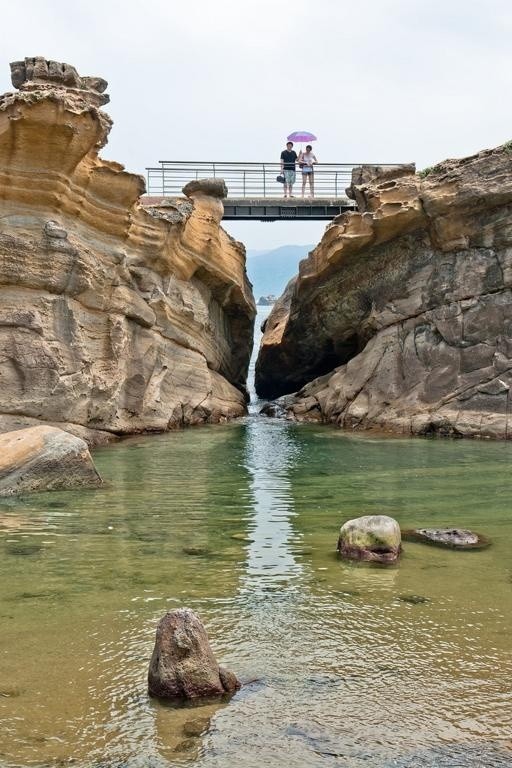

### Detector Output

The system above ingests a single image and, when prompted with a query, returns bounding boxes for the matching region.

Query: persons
[281,141,302,198]
[298,145,318,198]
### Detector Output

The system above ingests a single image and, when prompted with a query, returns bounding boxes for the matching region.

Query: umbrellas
[287,130,317,155]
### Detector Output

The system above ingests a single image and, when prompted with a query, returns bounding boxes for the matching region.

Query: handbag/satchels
[299,157,303,168]
[277,176,285,183]
